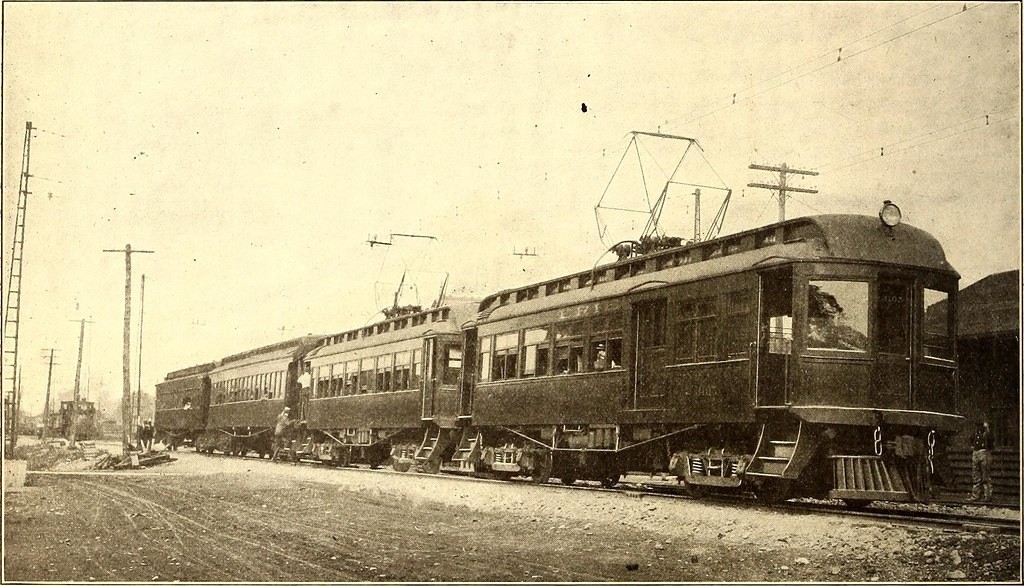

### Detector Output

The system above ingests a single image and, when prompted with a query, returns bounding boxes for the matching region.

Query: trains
[37,400,97,442]
[155,200,974,509]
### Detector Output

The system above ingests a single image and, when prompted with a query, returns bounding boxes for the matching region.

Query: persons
[137,420,154,449]
[809,302,859,351]
[965,418,994,502]
[268,406,295,462]
[555,354,569,373]
[593,344,615,369]
[183,400,192,410]
[297,367,311,421]
